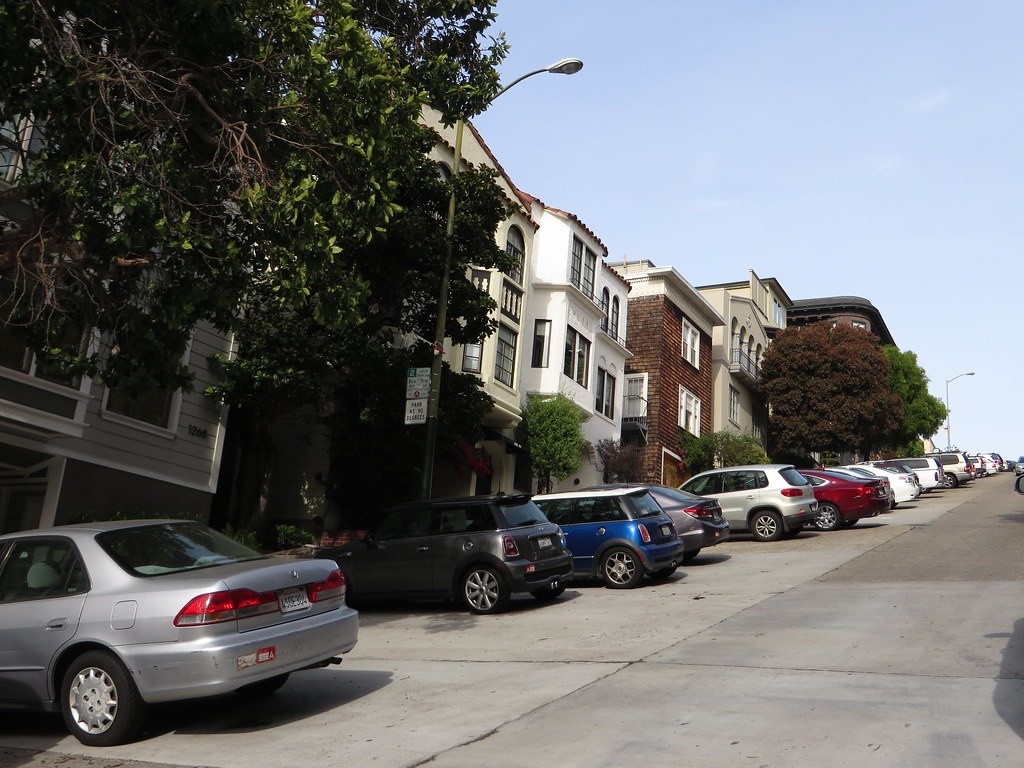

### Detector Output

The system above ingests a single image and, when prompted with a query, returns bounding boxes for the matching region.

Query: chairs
[26,557,61,598]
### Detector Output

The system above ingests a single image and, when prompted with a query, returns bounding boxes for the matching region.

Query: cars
[309,492,576,615]
[571,482,731,565]
[676,464,821,542]
[843,460,924,509]
[744,468,892,531]
[0,519,360,747]
[1014,456,1024,476]
[524,486,685,590]
[963,451,1016,479]
[809,466,895,510]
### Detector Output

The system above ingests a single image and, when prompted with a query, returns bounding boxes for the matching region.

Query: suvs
[885,456,946,494]
[919,451,977,489]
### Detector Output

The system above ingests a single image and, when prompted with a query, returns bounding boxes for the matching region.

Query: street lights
[420,57,584,501]
[946,371,976,453]
